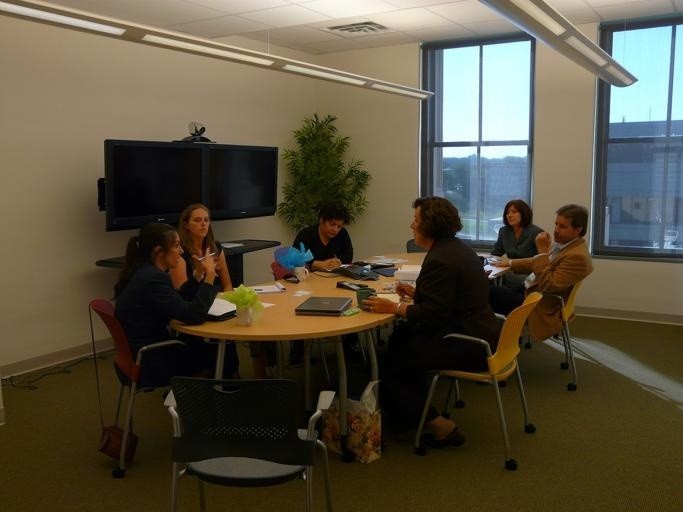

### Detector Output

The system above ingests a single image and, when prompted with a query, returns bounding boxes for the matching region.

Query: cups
[294,266,308,281]
[236,305,253,327]
[356,286,377,311]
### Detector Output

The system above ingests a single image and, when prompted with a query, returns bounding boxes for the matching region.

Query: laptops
[295,295,353,317]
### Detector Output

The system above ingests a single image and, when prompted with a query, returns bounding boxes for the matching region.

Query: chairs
[88,299,188,481]
[269,258,294,281]
[162,374,338,512]
[404,237,425,253]
[541,277,582,392]
[410,291,542,472]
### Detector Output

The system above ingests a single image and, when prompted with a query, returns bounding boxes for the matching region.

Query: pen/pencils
[198,252,216,262]
[334,254,336,258]
[254,289,262,290]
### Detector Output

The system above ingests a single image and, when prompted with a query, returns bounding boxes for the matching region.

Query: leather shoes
[418,426,468,450]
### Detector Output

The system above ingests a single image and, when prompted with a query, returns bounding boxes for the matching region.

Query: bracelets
[397,302,402,314]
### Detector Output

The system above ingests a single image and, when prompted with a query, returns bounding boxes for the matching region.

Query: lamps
[0,0,435,101]
[477,0,638,88]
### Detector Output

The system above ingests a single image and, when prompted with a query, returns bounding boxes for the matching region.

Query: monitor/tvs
[195,142,278,221]
[104,139,211,232]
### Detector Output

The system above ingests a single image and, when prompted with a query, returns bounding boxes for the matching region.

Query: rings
[369,306,373,312]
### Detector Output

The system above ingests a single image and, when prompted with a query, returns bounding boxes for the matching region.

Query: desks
[94,239,281,289]
[168,252,511,464]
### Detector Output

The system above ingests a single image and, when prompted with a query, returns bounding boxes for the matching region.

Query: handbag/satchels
[318,398,385,466]
[95,422,142,465]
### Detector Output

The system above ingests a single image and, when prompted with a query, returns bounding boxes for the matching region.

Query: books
[232,281,287,294]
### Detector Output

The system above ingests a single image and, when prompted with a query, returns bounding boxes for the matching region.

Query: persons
[360,196,501,449]
[287,206,364,365]
[489,200,544,288]
[110,223,217,387]
[169,203,240,392]
[488,205,592,345]
